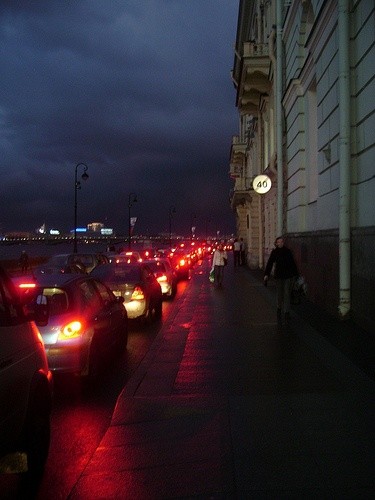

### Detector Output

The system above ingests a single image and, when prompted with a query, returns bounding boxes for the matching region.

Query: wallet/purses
[263,278,268,287]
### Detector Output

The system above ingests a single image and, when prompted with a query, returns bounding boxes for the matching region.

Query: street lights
[129,190,138,247]
[74,162,89,253]
[169,206,176,237]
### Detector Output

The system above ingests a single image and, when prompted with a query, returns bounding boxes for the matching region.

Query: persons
[210,237,246,288]
[19,249,29,272]
[263,237,299,321]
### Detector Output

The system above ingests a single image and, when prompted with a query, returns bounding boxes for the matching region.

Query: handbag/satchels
[223,256,228,267]
[289,277,301,306]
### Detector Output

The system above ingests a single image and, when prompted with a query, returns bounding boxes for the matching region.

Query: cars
[46,243,214,322]
[0,273,127,500]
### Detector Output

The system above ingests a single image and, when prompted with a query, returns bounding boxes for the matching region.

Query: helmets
[21,249,26,255]
